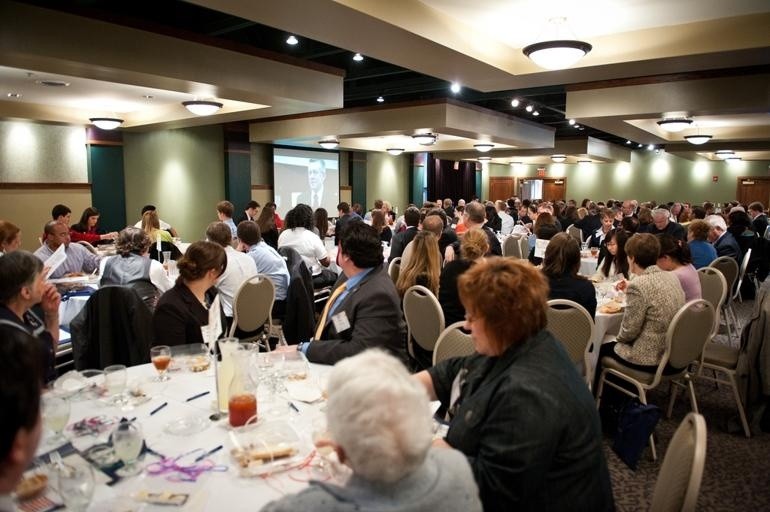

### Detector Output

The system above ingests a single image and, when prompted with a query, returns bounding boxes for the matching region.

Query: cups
[58,465,95,512]
[590,247,599,257]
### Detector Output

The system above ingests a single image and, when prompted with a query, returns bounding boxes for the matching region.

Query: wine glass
[150,344,173,383]
[581,241,588,258]
[111,422,146,478]
[104,366,127,407]
[41,393,73,448]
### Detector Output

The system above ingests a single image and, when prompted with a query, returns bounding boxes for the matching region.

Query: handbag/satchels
[609,397,662,473]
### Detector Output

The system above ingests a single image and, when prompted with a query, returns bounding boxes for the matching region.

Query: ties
[313,195,319,208]
[313,281,346,341]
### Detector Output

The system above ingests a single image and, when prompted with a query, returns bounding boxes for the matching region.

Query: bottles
[226,349,258,427]
[331,218,336,225]
[217,337,248,416]
[161,251,172,273]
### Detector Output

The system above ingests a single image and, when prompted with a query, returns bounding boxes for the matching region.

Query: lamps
[352,52,364,62]
[714,150,735,159]
[376,95,385,103]
[683,134,712,146]
[386,82,660,169]
[520,39,592,73]
[180,100,223,117]
[654,118,694,134]
[89,117,125,132]
[285,34,299,46]
[317,140,340,150]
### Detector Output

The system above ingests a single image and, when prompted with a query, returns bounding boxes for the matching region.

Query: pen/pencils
[92,267,97,275]
[150,402,168,415]
[194,446,223,462]
[290,403,300,412]
[187,392,210,401]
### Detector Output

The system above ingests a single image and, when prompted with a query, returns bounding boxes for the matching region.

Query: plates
[161,413,212,437]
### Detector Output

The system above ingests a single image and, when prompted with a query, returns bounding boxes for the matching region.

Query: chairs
[597,247,769,511]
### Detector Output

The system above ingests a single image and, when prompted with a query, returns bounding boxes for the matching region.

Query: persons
[259,347,485,511]
[30,188,769,435]
[0,324,44,511]
[0,219,22,257]
[410,256,618,511]
[0,251,61,383]
